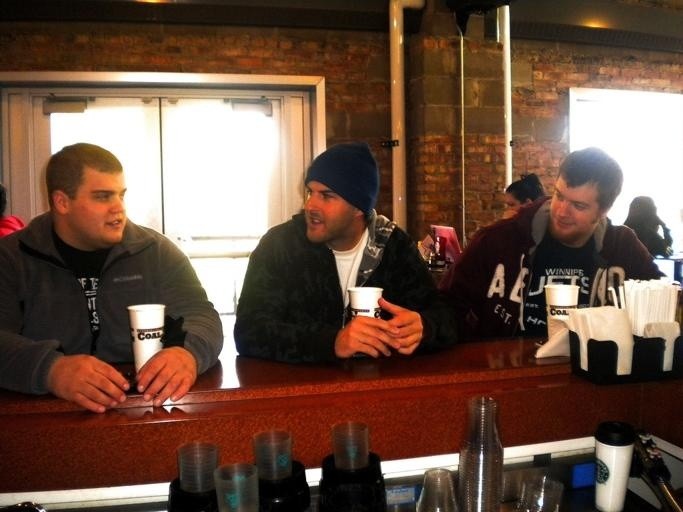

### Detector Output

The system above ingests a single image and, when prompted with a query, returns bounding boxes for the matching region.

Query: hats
[303,140,380,211]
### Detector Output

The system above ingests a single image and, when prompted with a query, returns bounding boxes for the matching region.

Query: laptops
[430,224,463,264]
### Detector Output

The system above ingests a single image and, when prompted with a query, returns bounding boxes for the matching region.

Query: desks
[655,257,683,284]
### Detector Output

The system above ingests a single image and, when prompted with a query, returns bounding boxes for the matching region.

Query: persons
[232,138,462,369]
[0,185,26,238]
[621,195,674,261]
[0,142,224,415]
[438,152,666,342]
[503,173,543,216]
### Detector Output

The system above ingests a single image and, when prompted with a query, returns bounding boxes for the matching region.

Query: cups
[348,287,383,361]
[545,284,579,346]
[171,417,390,512]
[593,419,636,511]
[123,302,171,388]
[416,395,564,512]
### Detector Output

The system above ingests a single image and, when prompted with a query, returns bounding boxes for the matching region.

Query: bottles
[427,251,445,266]
